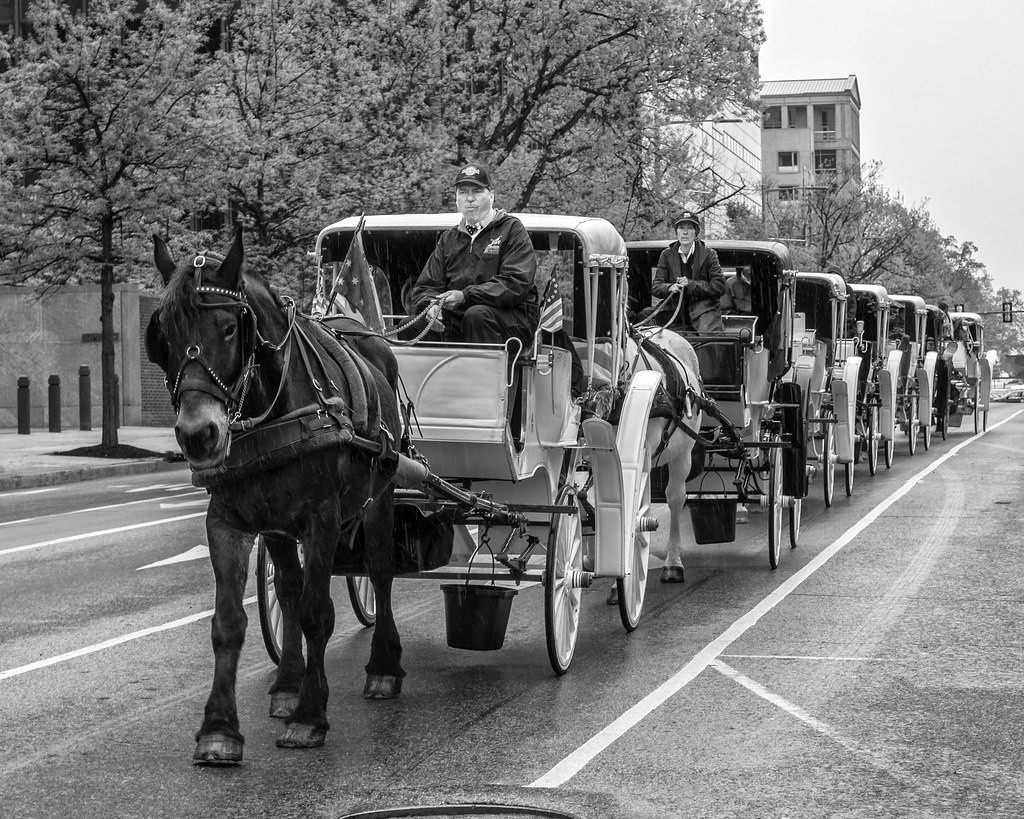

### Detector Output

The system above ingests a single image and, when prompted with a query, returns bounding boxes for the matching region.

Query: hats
[674,211,700,225]
[452,163,493,191]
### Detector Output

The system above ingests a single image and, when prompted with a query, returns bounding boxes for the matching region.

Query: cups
[856,321,864,333]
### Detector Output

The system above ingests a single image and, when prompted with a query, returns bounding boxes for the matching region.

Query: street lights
[653,112,743,207]
[761,183,831,238]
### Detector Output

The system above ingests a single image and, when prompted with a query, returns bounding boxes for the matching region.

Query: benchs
[382,315,542,473]
[637,310,764,407]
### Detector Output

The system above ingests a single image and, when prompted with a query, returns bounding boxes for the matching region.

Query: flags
[335,231,386,336]
[539,266,563,332]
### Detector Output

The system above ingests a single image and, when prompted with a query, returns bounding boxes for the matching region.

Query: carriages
[796,272,992,508]
[144,170,670,775]
[622,211,810,586]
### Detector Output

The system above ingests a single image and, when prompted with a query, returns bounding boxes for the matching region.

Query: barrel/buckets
[440,542,518,651]
[686,470,737,545]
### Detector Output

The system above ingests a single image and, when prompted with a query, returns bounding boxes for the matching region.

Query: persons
[722,266,751,311]
[824,266,857,338]
[397,163,541,351]
[938,303,949,314]
[651,210,729,338]
